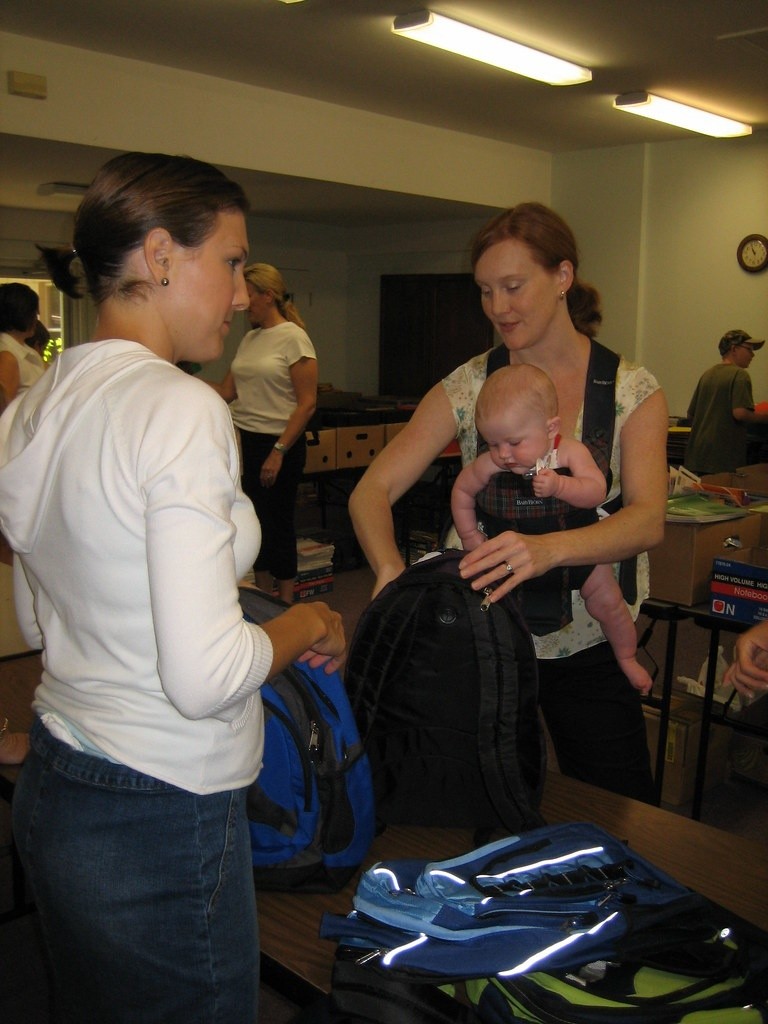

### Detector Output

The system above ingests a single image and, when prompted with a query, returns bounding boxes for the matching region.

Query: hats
[719,329,765,357]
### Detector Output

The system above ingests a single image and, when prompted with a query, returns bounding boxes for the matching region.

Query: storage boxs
[730,694,768,788]
[640,461,767,624]
[639,681,730,807]
[305,424,462,472]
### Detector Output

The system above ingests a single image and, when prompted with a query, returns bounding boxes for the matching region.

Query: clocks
[735,235,768,272]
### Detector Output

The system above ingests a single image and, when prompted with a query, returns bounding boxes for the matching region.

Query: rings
[268,474,272,478]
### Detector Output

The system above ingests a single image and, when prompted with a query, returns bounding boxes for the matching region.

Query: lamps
[36,181,89,199]
[393,8,595,90]
[613,92,754,141]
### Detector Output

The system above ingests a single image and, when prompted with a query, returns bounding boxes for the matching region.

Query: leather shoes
[1,718,30,765]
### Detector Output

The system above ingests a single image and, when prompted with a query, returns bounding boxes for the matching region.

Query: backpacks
[236,585,374,895]
[366,547,547,820]
[319,818,768,1024]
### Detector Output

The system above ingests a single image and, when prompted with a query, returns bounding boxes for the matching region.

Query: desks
[258,766,768,1024]
[302,402,457,566]
[617,599,768,823]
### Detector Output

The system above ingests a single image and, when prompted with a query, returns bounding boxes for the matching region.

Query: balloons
[505,560,514,574]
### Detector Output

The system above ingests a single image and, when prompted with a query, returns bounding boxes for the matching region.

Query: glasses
[731,343,753,351]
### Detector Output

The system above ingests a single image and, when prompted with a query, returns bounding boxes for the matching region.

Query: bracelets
[274,441,288,454]
[0,716,8,735]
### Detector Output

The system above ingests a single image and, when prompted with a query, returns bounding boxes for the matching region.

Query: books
[273,538,336,601]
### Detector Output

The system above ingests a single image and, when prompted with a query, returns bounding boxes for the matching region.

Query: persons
[449,362,655,696]
[348,201,669,809]
[0,149,349,1024]
[175,262,319,605]
[0,282,52,567]
[683,329,768,477]
[721,617,768,701]
[0,713,31,764]
